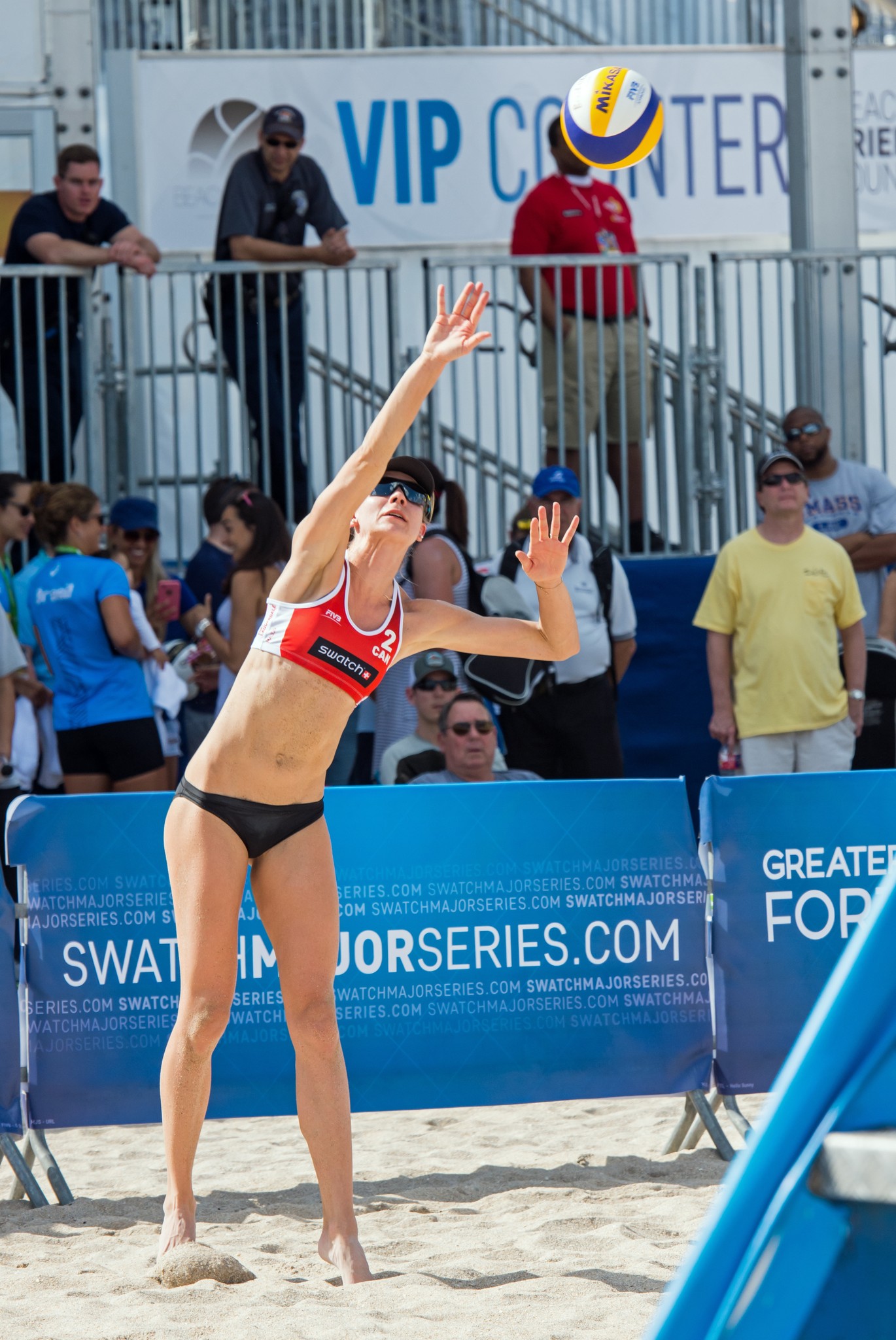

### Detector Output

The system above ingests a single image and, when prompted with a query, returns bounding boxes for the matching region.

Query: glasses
[265,137,299,149]
[122,529,159,543]
[370,476,427,513]
[9,501,31,518]
[763,472,809,487]
[444,720,494,737]
[416,676,458,692]
[786,421,822,443]
[90,514,106,526]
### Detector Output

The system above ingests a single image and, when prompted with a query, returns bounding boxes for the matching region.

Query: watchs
[1,754,14,777]
[847,689,867,702]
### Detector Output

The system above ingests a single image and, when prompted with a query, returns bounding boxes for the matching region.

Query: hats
[757,449,805,479]
[109,496,159,533]
[263,105,305,141]
[414,651,454,684]
[386,455,434,498]
[533,465,581,499]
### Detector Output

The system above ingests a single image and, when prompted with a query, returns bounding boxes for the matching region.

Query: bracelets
[193,617,213,640]
[644,315,652,327]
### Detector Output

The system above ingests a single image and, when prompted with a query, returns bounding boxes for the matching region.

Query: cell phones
[158,579,179,619]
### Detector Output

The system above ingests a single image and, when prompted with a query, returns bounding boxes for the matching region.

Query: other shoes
[616,519,681,555]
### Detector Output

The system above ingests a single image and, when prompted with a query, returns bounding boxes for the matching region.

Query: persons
[202,107,355,524]
[0,457,641,796]
[782,406,896,771]
[0,143,162,577]
[161,282,582,1288]
[508,115,681,553]
[691,451,867,774]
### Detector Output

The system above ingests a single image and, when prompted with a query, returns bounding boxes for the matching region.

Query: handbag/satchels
[464,574,558,706]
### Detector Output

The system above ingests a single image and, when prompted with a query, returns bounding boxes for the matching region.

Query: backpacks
[406,529,493,665]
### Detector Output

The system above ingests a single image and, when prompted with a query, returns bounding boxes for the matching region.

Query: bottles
[718,742,743,778]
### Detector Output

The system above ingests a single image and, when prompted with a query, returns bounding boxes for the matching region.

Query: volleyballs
[559,67,664,171]
